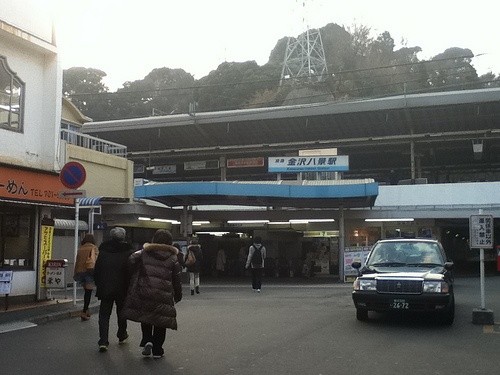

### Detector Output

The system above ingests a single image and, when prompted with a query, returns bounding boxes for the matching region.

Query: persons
[92,226,136,352]
[120,230,182,359]
[244,237,266,293]
[215,246,226,282]
[72,234,99,321]
[173,238,202,295]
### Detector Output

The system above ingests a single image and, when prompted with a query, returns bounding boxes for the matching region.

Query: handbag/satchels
[185,250,196,266]
[86,247,98,275]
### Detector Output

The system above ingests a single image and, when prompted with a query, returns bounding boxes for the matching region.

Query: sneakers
[151,346,167,358]
[99,344,108,352]
[118,336,128,344]
[142,341,152,358]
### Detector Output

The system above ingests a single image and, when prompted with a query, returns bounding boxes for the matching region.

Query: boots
[190,289,195,296]
[196,286,200,293]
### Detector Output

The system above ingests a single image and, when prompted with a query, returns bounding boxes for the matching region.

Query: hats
[154,229,173,245]
[254,236,262,244]
[109,226,126,241]
[191,237,199,245]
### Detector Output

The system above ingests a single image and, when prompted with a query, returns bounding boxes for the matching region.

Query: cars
[350,238,456,325]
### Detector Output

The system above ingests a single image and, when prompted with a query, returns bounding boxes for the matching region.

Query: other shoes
[257,289,261,293]
[252,289,256,292]
[80,311,88,321]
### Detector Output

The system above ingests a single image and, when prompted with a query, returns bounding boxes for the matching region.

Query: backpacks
[251,245,265,267]
